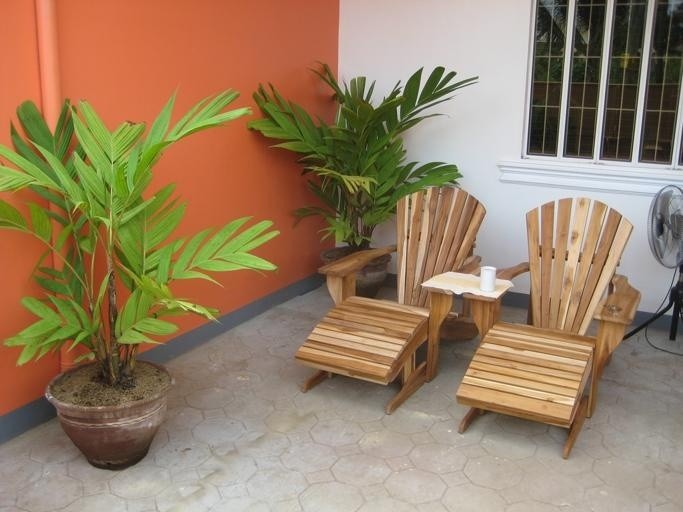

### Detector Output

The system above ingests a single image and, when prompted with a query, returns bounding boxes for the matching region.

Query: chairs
[456,197,641,459]
[294,186,486,415]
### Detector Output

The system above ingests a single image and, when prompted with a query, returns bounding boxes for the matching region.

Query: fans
[620,183,683,342]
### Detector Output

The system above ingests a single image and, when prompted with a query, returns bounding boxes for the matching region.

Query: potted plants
[0,84,280,470]
[247,59,479,298]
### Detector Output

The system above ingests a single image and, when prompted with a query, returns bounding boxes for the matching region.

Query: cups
[479,265,496,292]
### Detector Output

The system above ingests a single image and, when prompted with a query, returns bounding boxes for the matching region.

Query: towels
[420,270,514,301]
